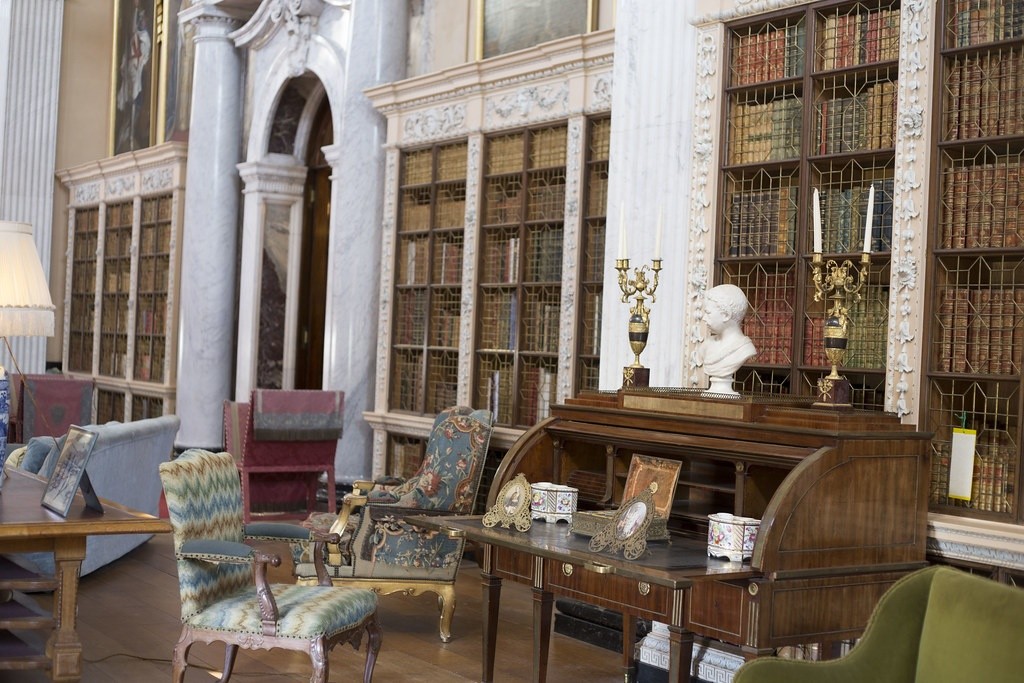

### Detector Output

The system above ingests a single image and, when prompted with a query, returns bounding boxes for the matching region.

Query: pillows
[6,435,60,476]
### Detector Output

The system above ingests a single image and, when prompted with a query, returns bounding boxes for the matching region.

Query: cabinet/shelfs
[384,107,611,553]
[66,191,175,425]
[712,1,1024,525]
[405,390,935,683]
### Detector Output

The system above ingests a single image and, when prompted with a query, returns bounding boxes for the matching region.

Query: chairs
[288,404,495,646]
[156,448,384,683]
[223,388,346,524]
[7,370,95,443]
[732,561,1024,682]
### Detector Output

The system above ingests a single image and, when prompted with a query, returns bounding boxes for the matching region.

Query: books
[929,0,1024,518]
[392,119,611,426]
[724,5,899,369]
[69,195,172,425]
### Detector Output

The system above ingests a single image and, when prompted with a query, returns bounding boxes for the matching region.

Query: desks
[0,461,175,683]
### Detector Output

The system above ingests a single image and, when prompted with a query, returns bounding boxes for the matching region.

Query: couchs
[0,412,183,580]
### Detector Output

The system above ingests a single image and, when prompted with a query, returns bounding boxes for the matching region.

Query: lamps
[0,219,56,474]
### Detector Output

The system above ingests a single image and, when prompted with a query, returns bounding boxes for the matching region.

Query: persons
[505,486,520,515]
[701,284,757,395]
[116,6,151,153]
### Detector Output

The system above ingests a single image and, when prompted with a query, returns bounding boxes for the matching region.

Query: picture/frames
[475,0,599,62]
[107,0,195,157]
[39,423,100,517]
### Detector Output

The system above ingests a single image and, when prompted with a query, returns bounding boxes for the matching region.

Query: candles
[812,186,822,252]
[653,212,663,260]
[863,184,874,252]
[616,200,629,259]
